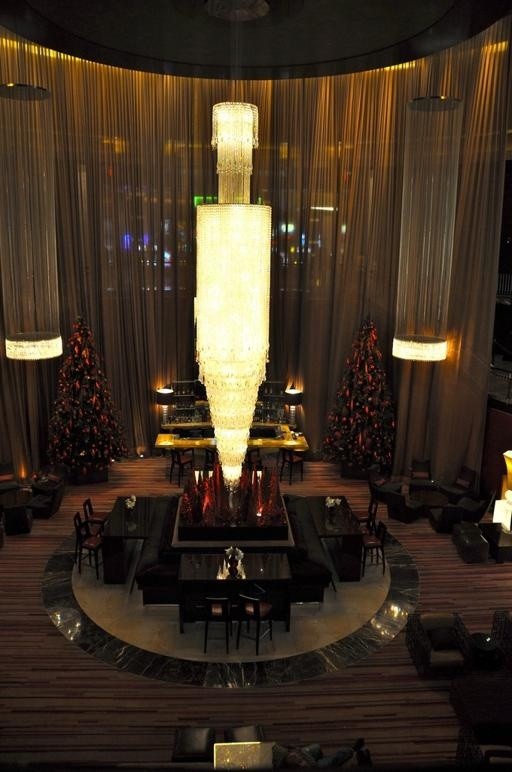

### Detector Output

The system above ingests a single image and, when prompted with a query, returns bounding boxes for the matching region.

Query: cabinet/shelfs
[171,381,287,420]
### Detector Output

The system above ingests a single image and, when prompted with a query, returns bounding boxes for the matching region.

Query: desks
[101,495,363,632]
[155,433,309,468]
[161,419,290,435]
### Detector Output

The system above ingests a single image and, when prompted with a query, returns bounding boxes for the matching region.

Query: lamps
[393,94,461,362]
[157,389,174,424]
[193,8,272,494]
[284,390,302,426]
[0,84,63,362]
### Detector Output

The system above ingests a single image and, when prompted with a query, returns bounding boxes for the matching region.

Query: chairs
[76,496,105,580]
[362,500,386,572]
[173,721,374,769]
[201,592,276,656]
[0,463,63,548]
[407,607,510,770]
[367,456,495,534]
[169,447,303,491]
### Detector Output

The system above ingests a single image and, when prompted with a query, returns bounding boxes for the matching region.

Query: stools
[450,523,489,563]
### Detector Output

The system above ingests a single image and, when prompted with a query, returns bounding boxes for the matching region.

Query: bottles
[173,384,272,423]
